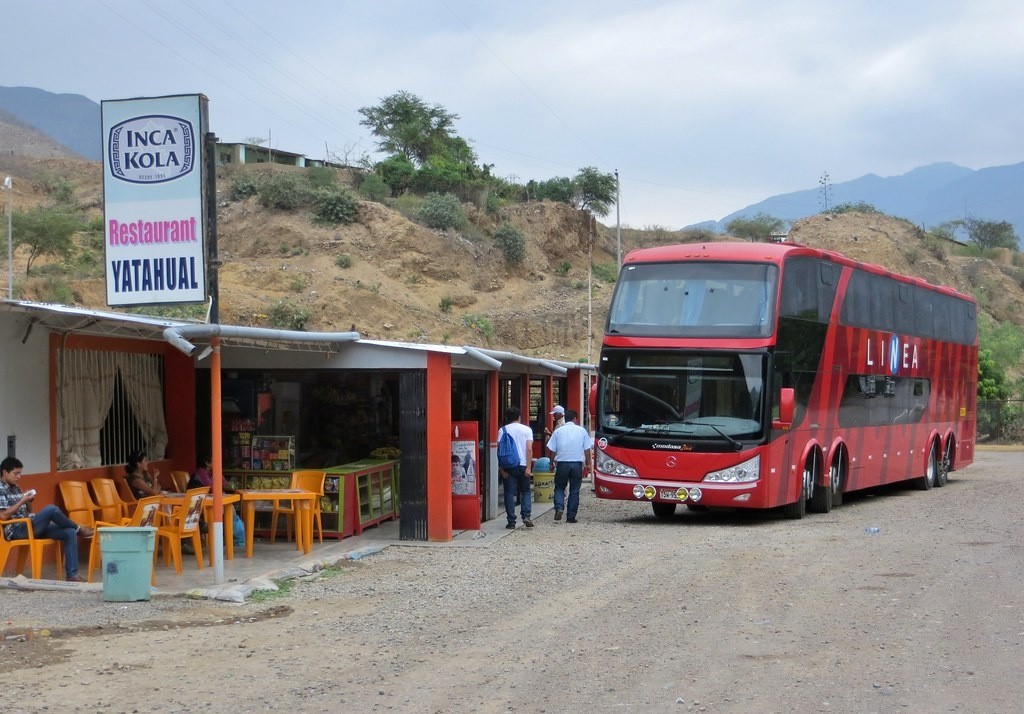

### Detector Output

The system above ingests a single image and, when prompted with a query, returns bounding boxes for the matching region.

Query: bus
[589,241,978,521]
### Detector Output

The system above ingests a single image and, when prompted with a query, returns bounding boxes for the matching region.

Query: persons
[124,451,195,555]
[450,455,466,484]
[0,457,95,582]
[186,454,247,546]
[544,405,592,523]
[498,407,534,529]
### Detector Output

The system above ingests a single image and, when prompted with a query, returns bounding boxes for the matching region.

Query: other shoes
[554,510,563,520]
[182,544,195,555]
[523,517,534,527]
[506,521,516,529]
[200,523,209,533]
[566,520,578,523]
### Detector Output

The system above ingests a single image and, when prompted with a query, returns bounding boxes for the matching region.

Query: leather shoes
[66,575,88,582]
[76,527,94,536]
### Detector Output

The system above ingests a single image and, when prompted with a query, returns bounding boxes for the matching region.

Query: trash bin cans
[97,526,157,603]
[532,458,557,503]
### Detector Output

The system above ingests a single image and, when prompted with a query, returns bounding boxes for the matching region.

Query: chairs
[0,471,326,586]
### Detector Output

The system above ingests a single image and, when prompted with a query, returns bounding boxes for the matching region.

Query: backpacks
[496,426,521,470]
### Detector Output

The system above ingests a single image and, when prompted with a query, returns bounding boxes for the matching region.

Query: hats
[549,406,565,415]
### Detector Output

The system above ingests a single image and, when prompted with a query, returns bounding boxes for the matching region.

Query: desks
[161,494,239,567]
[236,488,316,558]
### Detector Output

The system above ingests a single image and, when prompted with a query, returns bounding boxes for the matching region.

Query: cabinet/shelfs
[224,380,274,469]
[251,435,295,470]
[223,469,354,541]
[329,459,400,535]
